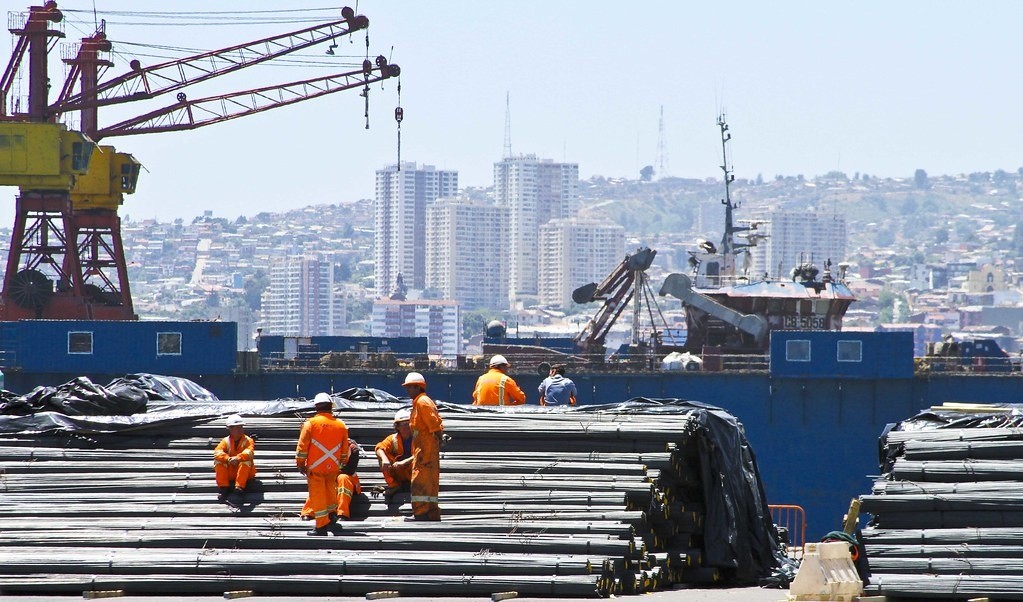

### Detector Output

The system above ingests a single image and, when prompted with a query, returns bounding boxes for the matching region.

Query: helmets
[393,409,411,422]
[314,392,335,407]
[489,354,511,368]
[226,414,246,426]
[336,418,349,431]
[402,373,426,386]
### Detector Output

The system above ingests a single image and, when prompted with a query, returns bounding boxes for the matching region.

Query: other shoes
[308,527,327,536]
[404,514,428,521]
[218,489,227,500]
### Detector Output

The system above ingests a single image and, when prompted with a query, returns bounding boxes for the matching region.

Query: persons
[301,438,361,520]
[401,371,451,521]
[473,354,524,406]
[213,414,255,504]
[296,393,348,536]
[538,365,578,407]
[375,410,412,496]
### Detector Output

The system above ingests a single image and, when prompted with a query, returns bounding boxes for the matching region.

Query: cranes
[0,0,402,321]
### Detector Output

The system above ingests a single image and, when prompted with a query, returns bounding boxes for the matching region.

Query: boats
[480,86,860,374]
